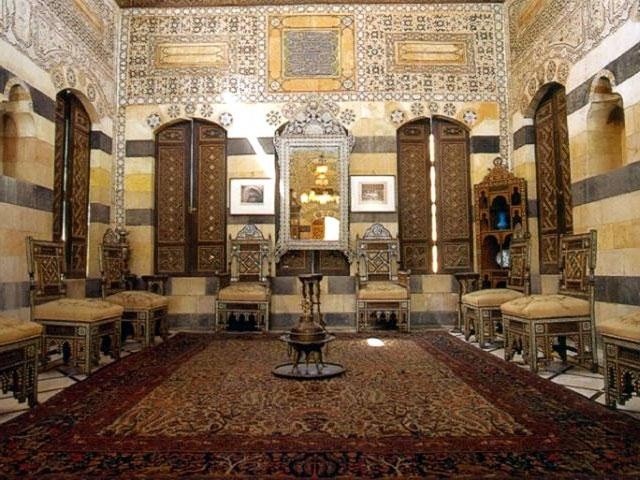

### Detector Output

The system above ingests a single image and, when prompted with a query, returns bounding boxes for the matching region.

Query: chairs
[20,234,170,380]
[213,245,273,332]
[355,240,412,335]
[457,230,640,413]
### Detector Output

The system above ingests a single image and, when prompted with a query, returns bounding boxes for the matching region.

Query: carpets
[0,330,640,478]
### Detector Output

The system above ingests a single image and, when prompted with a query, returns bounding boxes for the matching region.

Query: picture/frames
[350,174,399,214]
[229,178,275,215]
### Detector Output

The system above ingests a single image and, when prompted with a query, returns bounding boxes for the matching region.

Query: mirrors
[273,128,351,254]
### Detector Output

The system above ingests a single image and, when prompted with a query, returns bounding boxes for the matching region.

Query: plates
[495,250,509,266]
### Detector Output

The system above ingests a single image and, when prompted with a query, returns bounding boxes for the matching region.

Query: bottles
[496,212,507,229]
